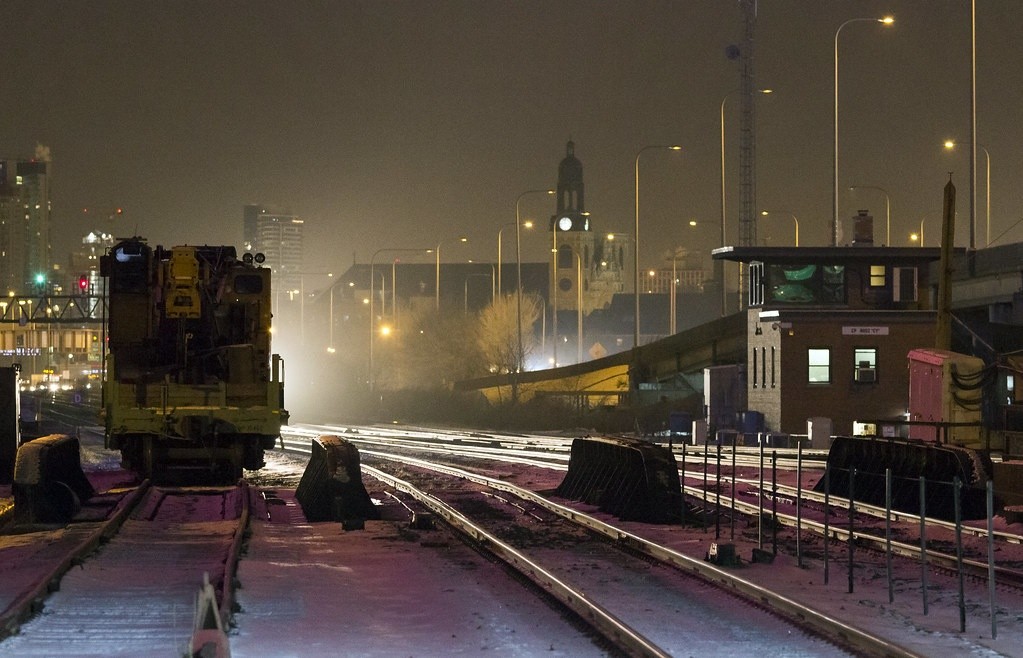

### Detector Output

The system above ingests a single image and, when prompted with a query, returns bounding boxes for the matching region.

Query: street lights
[498,221,534,299]
[762,210,798,246]
[436,238,466,307]
[720,87,773,318]
[553,208,590,368]
[552,248,583,363]
[516,190,556,373]
[370,249,434,382]
[848,185,889,245]
[834,17,896,245]
[690,220,743,312]
[469,257,495,306]
[947,141,990,247]
[633,145,682,346]
[393,250,437,318]
[920,210,958,246]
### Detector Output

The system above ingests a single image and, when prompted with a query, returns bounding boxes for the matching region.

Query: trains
[100,234,289,483]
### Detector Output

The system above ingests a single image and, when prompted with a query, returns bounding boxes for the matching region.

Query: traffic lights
[37,271,44,288]
[80,274,87,290]
